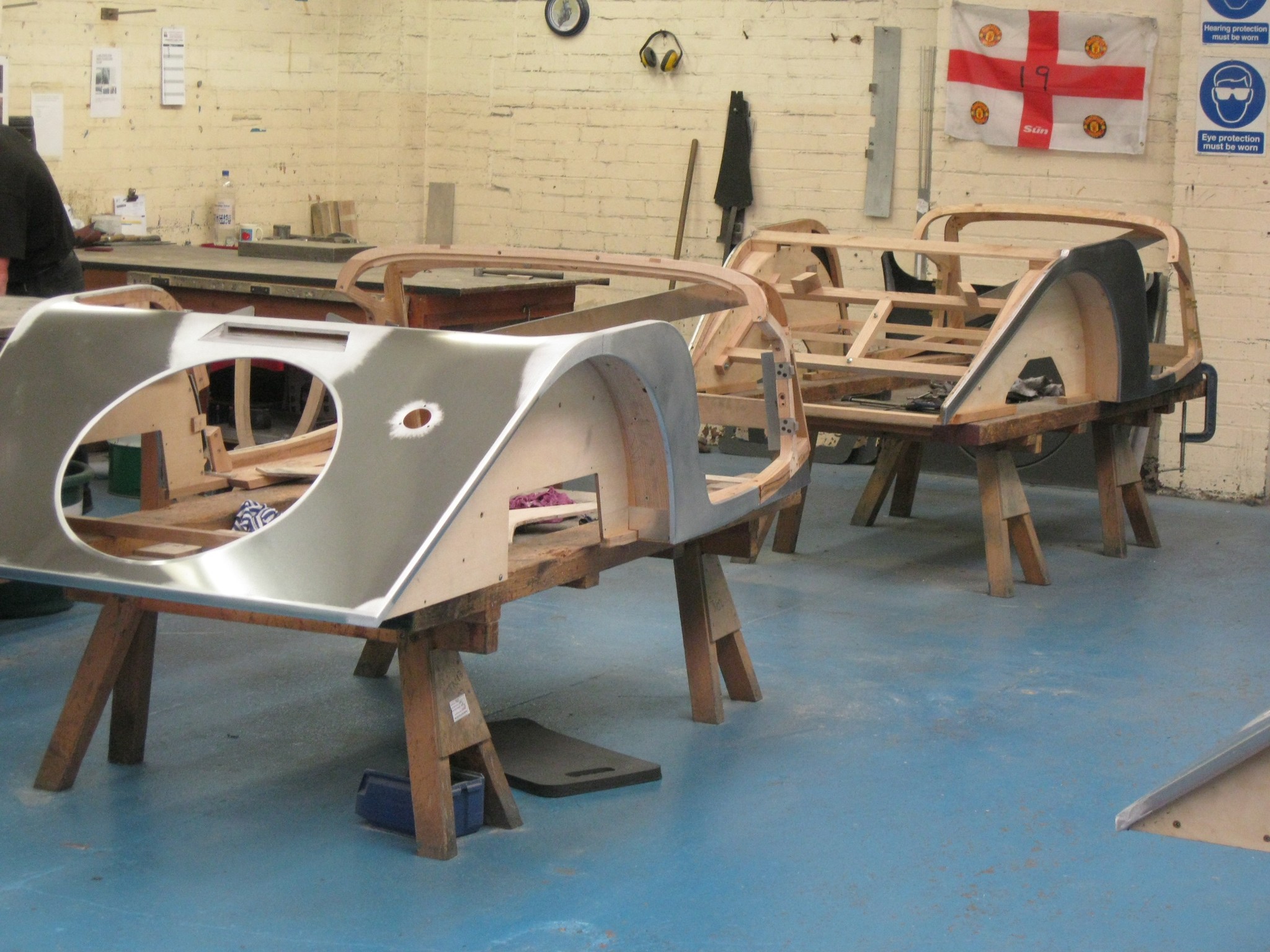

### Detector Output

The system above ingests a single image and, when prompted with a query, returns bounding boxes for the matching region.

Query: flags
[947,2,1158,159]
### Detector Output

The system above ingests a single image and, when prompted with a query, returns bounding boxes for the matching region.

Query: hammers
[472,263,567,280]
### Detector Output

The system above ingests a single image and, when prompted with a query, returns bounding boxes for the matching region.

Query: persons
[0,121,95,514]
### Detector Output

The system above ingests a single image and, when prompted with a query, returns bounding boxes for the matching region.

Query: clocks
[544,0,589,36]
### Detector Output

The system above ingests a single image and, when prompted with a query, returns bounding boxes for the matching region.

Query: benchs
[680,349,1219,596]
[10,490,796,864]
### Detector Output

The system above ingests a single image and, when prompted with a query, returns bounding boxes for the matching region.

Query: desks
[75,239,614,329]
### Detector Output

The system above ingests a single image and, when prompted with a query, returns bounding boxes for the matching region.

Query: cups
[239,224,263,242]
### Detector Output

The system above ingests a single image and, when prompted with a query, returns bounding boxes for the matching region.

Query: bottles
[213,170,235,246]
